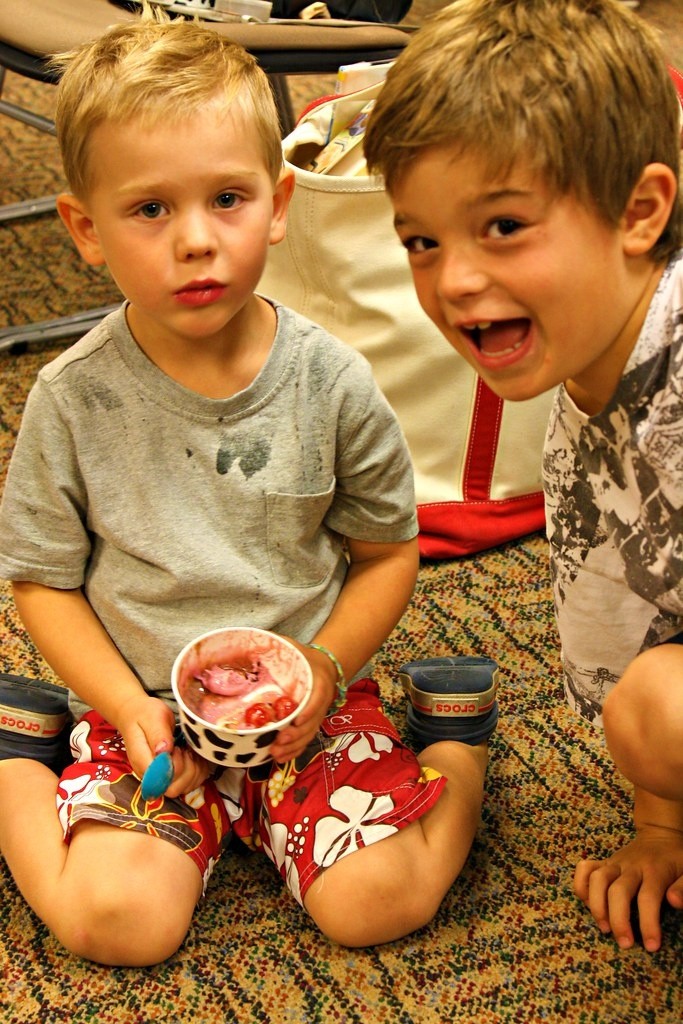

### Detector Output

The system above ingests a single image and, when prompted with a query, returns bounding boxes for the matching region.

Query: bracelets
[309,642,346,716]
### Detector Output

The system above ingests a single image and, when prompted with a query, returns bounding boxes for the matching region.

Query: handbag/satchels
[255,87,561,558]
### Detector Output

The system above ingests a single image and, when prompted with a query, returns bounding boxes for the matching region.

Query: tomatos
[274,697,298,720]
[245,703,273,726]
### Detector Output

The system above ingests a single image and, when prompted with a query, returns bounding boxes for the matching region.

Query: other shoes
[397,656,500,753]
[0,673,69,780]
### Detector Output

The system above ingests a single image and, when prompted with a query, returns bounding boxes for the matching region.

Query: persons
[0,26,497,967]
[363,0,683,952]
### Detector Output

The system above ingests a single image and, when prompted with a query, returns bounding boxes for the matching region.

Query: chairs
[0,0,419,358]
